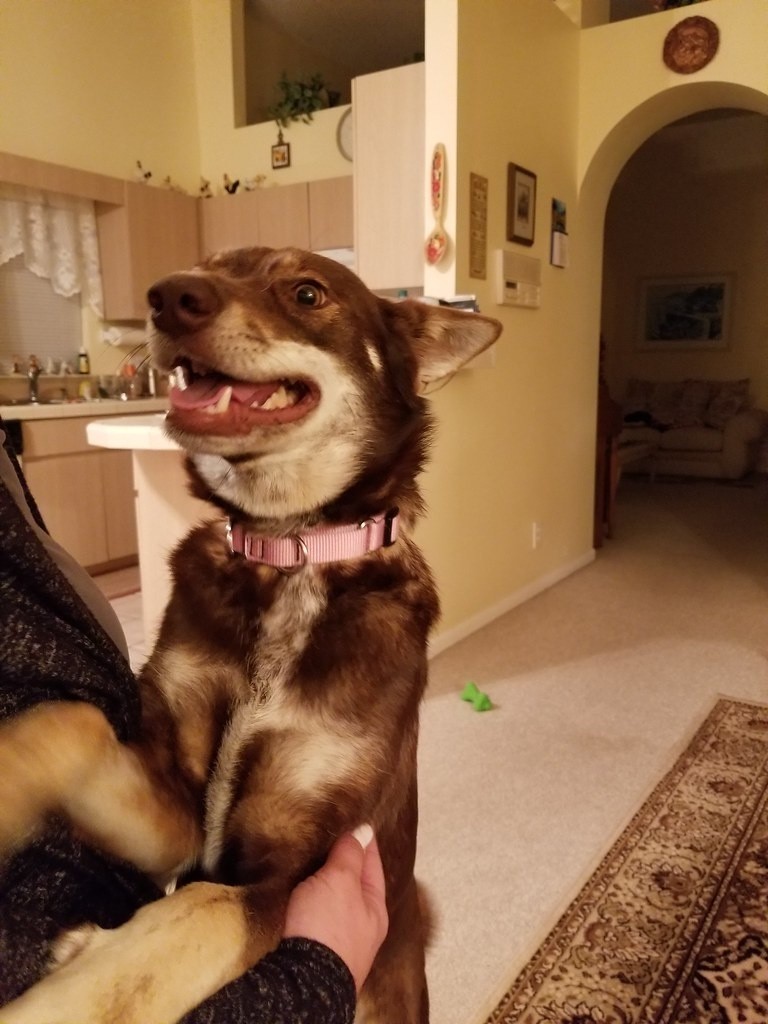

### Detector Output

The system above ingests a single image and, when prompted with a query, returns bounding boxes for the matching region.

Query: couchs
[623,409,768,483]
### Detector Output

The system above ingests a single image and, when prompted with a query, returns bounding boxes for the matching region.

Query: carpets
[474,694,768,1024]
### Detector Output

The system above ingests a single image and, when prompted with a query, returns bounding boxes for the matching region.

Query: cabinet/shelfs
[95,174,352,320]
[17,415,138,576]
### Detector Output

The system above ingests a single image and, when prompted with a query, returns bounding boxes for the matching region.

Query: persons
[0,429,388,1024]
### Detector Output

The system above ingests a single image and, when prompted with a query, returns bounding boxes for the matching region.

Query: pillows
[625,377,751,429]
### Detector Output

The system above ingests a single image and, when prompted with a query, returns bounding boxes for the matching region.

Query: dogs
[0,242,506,1024]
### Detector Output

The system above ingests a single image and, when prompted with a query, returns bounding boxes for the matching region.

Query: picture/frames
[507,161,537,246]
[637,271,732,353]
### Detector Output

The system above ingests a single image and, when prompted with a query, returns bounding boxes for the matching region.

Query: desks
[618,439,660,483]
